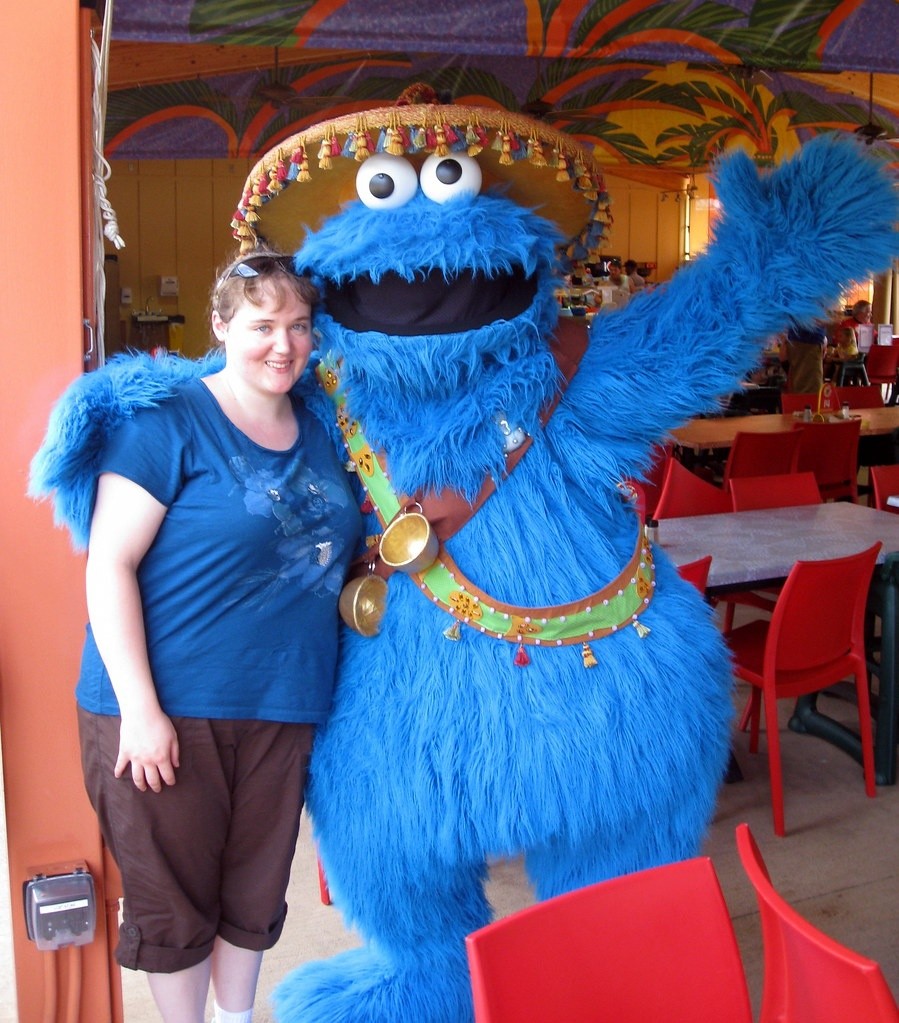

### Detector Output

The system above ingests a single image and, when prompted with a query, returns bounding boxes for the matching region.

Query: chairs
[677,540,883,832]
[777,333,899,413]
[637,415,899,634]
[465,823,899,1023]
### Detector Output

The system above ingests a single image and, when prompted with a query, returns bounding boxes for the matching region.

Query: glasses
[229,256,296,278]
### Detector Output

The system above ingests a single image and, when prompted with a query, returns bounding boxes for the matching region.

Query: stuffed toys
[25,81,899,1023]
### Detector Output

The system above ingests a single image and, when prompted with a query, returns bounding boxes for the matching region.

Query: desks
[669,406,898,486]
[639,501,899,789]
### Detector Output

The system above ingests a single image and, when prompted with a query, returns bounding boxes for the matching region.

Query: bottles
[842,402,849,418]
[646,518,660,544]
[804,405,812,421]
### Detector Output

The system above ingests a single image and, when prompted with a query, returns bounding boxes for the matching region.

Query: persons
[842,300,878,355]
[74,250,365,1023]
[779,325,827,393]
[561,259,646,308]
[825,327,859,383]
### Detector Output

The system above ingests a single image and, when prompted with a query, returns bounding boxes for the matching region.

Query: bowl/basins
[378,504,439,573]
[338,574,388,637]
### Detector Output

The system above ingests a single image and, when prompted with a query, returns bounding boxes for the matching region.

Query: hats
[623,260,637,267]
